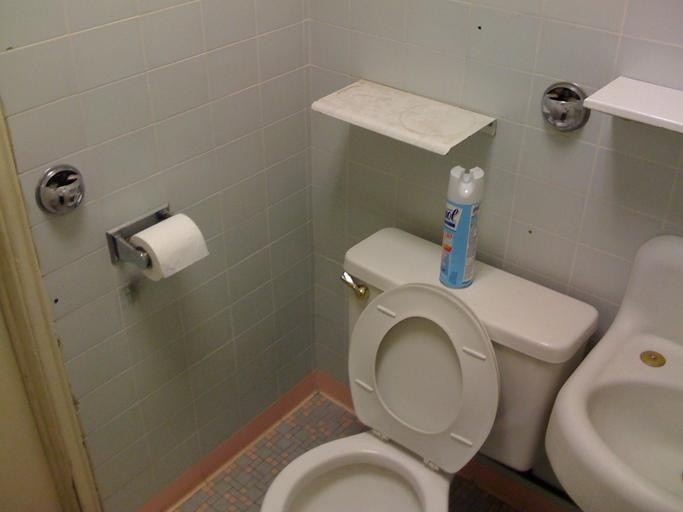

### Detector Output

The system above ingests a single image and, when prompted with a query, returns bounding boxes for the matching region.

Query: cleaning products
[439,164,484,289]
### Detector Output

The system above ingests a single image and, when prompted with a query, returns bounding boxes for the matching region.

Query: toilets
[258,226,598,512]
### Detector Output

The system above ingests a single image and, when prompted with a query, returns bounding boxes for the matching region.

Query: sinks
[545,333,683,512]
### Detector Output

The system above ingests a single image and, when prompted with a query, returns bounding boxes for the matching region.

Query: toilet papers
[129,213,210,283]
